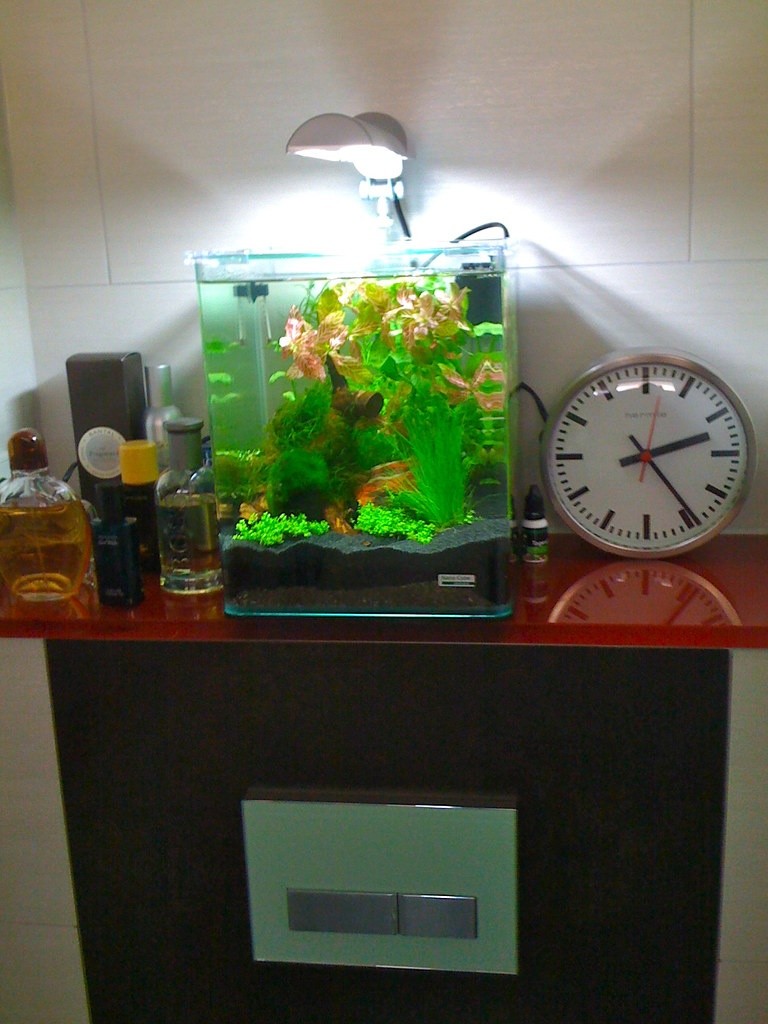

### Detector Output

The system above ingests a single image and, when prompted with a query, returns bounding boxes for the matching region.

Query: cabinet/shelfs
[1,533,768,1022]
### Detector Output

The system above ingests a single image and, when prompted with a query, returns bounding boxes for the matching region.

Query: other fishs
[204,283,503,556]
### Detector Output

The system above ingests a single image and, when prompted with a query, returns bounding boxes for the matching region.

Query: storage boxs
[192,240,520,617]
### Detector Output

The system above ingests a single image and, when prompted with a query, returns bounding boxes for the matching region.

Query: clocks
[549,561,742,626]
[540,344,756,561]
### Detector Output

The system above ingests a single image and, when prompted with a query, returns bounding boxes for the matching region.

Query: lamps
[284,110,408,228]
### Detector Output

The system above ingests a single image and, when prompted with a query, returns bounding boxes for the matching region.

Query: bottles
[116,439,163,574]
[509,484,549,565]
[89,482,144,608]
[151,417,224,596]
[0,426,90,603]
[143,362,184,446]
